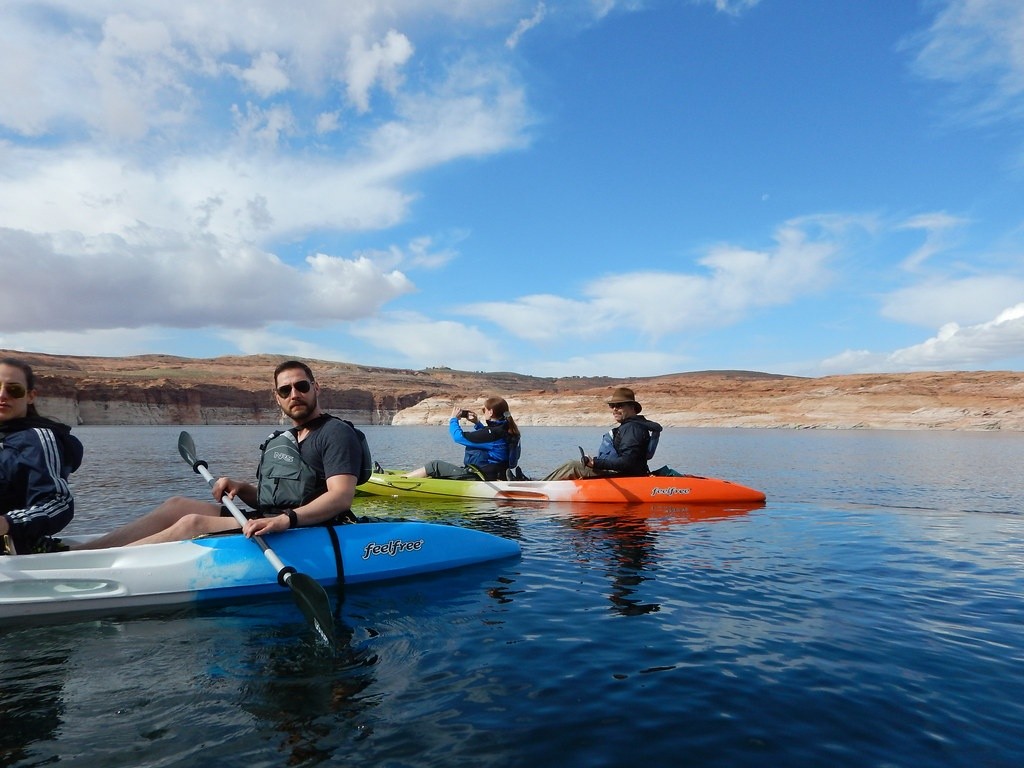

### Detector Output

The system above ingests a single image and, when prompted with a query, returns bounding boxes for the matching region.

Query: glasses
[0,383,32,399]
[275,380,315,399]
[482,408,491,413]
[609,403,628,409]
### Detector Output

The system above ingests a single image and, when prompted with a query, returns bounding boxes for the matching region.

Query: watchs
[282,509,297,530]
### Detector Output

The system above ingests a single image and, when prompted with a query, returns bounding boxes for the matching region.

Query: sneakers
[4,532,69,555]
[506,466,532,481]
[373,461,387,474]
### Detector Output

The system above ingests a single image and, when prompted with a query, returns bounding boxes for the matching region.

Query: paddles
[578,445,590,470]
[177,430,339,660]
[466,463,487,482]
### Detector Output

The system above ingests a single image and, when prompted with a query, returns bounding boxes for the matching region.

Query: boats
[353,468,768,504]
[0,515,523,620]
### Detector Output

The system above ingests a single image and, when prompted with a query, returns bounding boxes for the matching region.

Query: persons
[370,396,522,481]
[42,361,371,551]
[0,356,84,557]
[505,386,650,481]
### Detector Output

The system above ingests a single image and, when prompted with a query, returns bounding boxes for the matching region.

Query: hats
[607,388,642,414]
